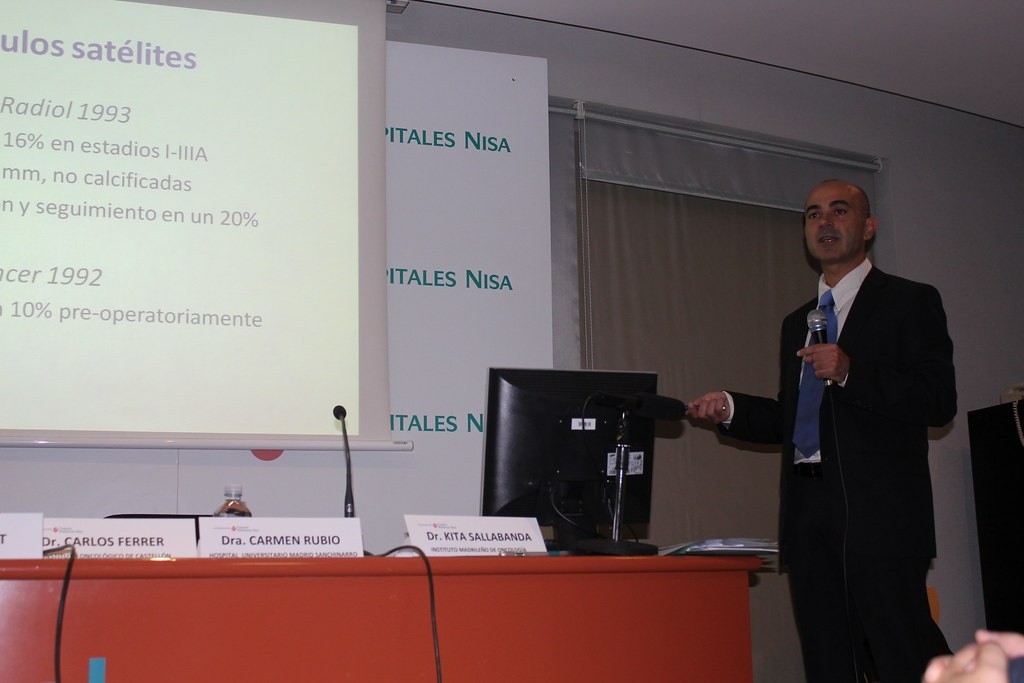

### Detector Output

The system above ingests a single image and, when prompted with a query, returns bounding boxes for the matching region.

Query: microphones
[592,390,686,422]
[333,405,355,517]
[807,310,834,389]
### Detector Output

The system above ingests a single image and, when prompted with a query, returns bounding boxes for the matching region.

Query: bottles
[213,484,252,517]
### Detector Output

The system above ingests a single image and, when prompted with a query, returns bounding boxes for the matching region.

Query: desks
[0,553,764,683]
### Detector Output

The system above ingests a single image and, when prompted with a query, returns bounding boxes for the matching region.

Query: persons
[687,179,959,683]
[921,629,1024,683]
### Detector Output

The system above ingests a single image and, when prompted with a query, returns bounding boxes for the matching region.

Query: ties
[791,289,838,458]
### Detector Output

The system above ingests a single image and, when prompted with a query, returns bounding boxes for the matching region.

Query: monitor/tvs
[479,367,658,556]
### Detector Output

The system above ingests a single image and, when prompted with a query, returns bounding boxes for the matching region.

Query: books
[659,538,780,556]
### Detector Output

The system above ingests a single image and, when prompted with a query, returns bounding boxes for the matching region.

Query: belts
[792,462,824,480]
